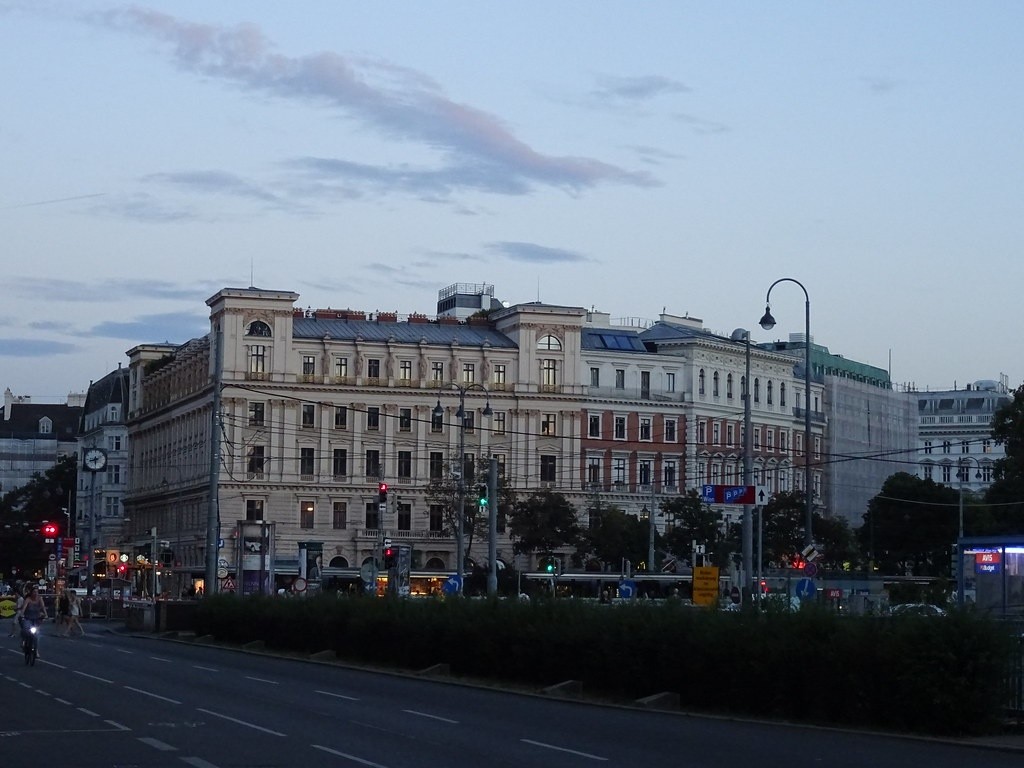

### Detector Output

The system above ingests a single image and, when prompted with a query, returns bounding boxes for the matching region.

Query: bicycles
[18,615,47,667]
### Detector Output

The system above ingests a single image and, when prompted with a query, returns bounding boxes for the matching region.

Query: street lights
[759,278,814,564]
[433,381,493,594]
[730,328,756,617]
[954,456,984,606]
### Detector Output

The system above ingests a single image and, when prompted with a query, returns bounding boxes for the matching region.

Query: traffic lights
[379,483,387,503]
[42,523,59,537]
[477,484,489,508]
[385,549,393,570]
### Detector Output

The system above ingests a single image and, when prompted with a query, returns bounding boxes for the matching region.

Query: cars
[876,601,946,618]
[726,591,800,616]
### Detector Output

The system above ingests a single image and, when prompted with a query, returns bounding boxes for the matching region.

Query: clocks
[81,448,108,473]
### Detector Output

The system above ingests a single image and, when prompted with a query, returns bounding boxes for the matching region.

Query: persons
[57,590,88,637]
[0,578,48,657]
[155,584,202,601]
[310,556,322,579]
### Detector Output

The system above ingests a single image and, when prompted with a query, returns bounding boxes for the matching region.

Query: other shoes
[36,649,40,658]
[57,632,61,636]
[21,641,25,647]
[80,632,85,637]
[8,634,15,638]
[61,634,70,637]
